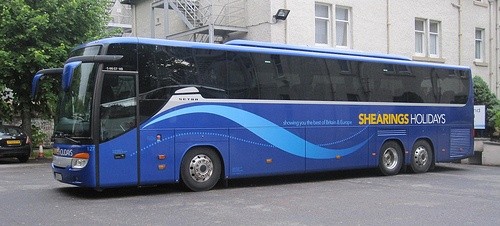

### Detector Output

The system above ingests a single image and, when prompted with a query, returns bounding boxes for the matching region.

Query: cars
[0,125,30,163]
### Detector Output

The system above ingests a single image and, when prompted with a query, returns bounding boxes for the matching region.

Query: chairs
[273,83,454,104]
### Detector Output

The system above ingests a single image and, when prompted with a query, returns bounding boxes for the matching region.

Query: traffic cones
[35,144,47,160]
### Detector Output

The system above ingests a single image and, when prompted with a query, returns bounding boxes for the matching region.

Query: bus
[28,37,476,192]
